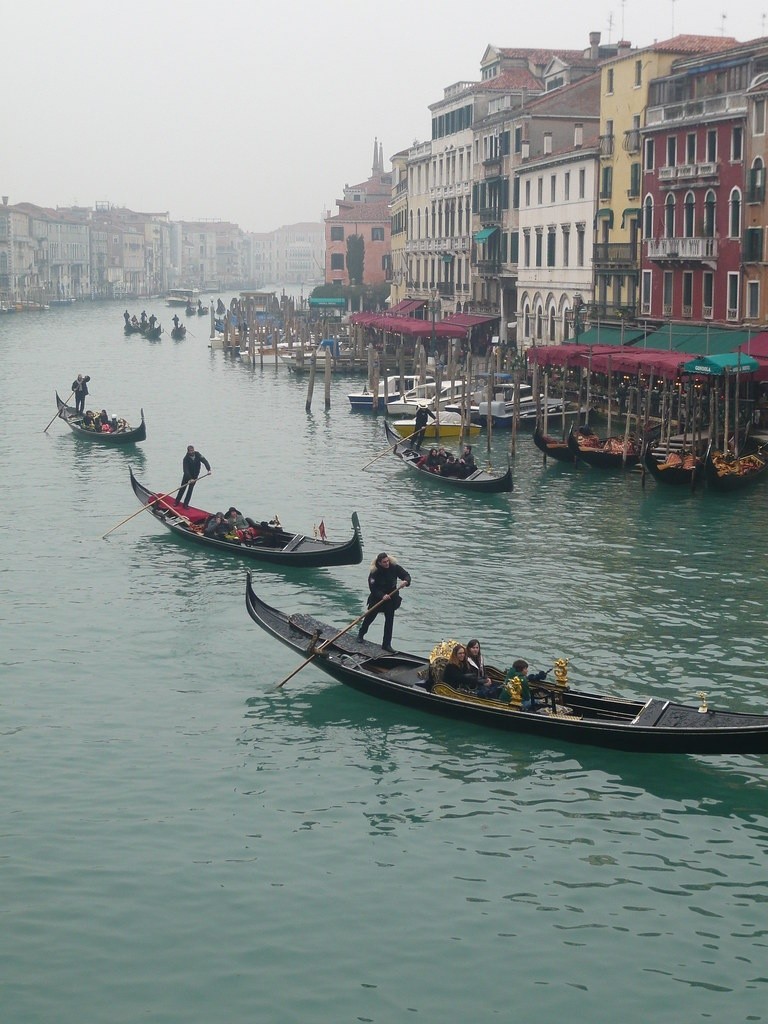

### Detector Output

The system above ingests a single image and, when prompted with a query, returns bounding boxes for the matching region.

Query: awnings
[474,226,499,243]
[559,323,768,359]
[386,299,427,315]
[440,314,499,327]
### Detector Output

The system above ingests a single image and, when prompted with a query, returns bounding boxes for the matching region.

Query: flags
[655,218,665,249]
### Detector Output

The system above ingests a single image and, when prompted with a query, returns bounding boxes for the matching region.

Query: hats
[418,402,428,408]
[112,414,117,418]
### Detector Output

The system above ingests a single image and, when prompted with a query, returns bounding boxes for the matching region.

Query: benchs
[416,638,467,698]
[205,509,260,543]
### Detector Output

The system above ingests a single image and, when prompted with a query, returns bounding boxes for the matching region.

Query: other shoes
[382,642,396,653]
[173,503,179,506]
[183,504,190,509]
[357,628,366,642]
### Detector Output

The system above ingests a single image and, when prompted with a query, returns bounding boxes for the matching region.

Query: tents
[527,343,768,425]
[349,312,466,357]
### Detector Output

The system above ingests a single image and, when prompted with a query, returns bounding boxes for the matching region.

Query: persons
[172,314,179,328]
[228,507,249,530]
[83,410,117,431]
[186,299,202,309]
[173,446,211,508]
[124,310,157,329]
[208,512,234,541]
[499,660,540,709]
[409,403,437,451]
[425,445,474,479]
[442,639,502,697]
[357,552,411,653]
[72,374,90,414]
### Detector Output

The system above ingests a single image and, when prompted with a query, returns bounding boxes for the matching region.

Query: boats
[55,388,146,444]
[128,464,365,567]
[347,375,768,494]
[124,288,208,338]
[0,299,77,313]
[209,290,378,372]
[244,566,768,755]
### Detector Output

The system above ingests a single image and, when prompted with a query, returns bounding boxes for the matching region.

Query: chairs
[737,455,751,473]
[241,530,254,545]
[528,685,574,716]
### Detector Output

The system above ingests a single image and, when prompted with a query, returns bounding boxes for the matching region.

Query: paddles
[578,446,601,452]
[362,420,437,471]
[150,492,205,535]
[716,466,736,478]
[44,376,86,432]
[657,456,701,470]
[178,322,196,338]
[276,585,403,689]
[155,320,170,336]
[103,473,211,538]
[546,443,567,448]
